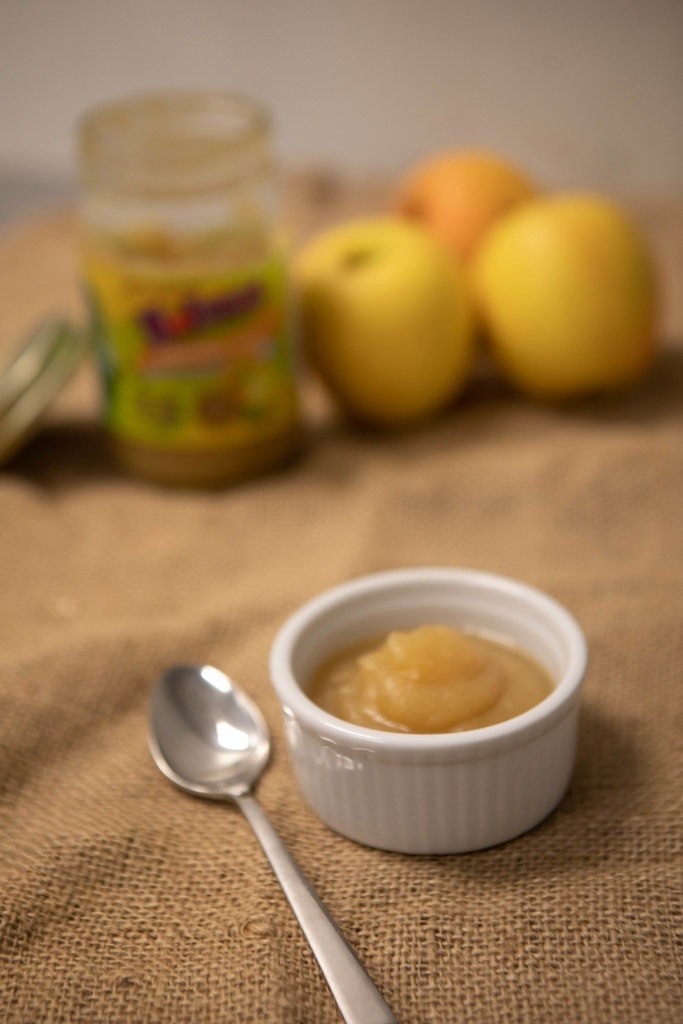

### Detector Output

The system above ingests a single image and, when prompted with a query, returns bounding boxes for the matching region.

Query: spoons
[144,663,398,1024]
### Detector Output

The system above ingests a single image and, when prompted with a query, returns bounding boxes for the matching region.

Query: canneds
[71,93,314,489]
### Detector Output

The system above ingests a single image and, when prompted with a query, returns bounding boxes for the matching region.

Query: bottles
[70,86,311,486]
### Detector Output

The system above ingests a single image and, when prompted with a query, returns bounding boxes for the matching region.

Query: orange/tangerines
[288,149,658,429]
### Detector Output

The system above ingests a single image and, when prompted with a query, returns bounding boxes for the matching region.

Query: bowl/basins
[267,565,588,854]
